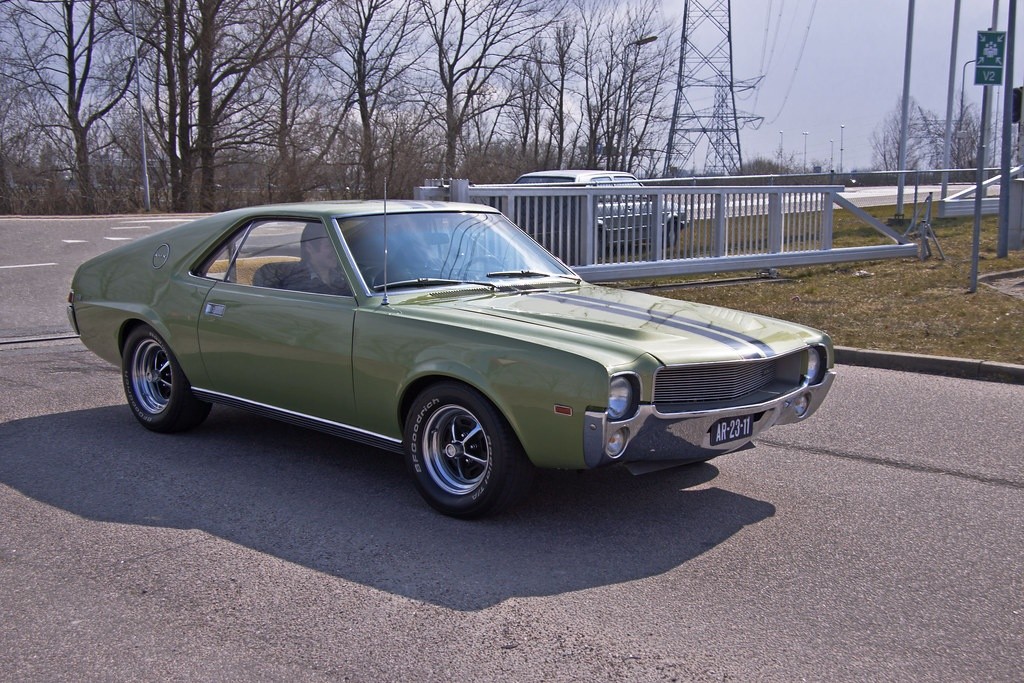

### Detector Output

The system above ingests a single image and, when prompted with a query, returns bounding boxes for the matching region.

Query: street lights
[623,35,660,172]
[802,131,809,173]
[830,139,834,171]
[779,131,784,173]
[840,125,845,172]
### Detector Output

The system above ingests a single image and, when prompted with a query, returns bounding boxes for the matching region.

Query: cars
[506,169,693,256]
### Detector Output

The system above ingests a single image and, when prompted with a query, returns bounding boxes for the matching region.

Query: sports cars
[63,199,838,524]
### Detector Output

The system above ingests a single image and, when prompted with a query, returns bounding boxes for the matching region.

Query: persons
[375,220,459,286]
[274,223,352,296]
